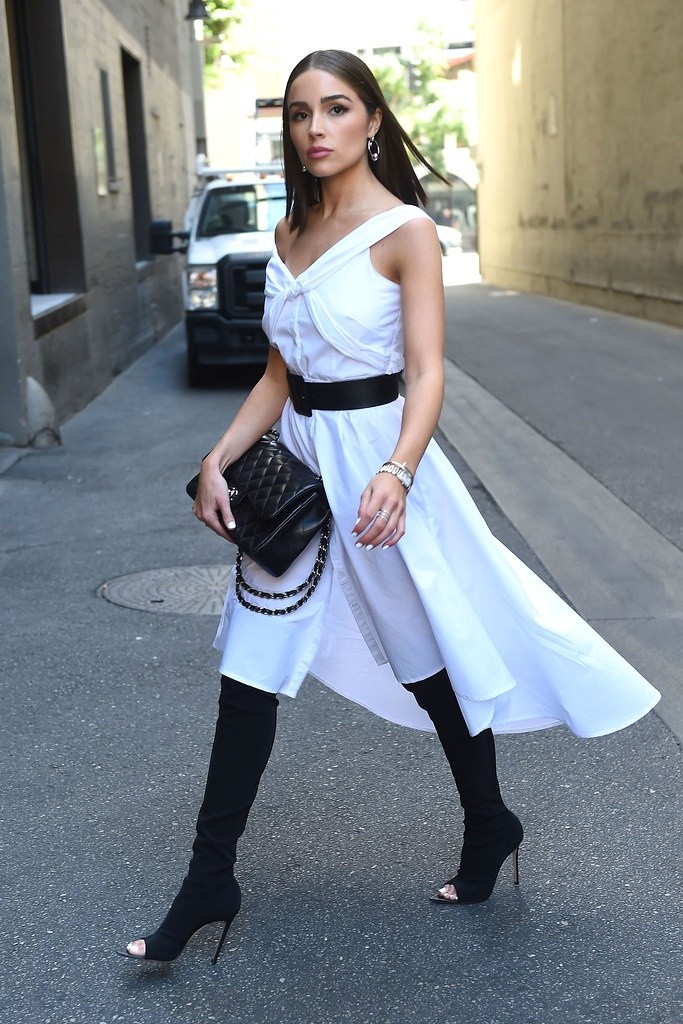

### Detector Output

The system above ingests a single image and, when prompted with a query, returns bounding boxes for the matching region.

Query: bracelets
[382,460,414,486]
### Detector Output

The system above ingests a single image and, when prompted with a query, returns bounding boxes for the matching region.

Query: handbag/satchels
[186,429,333,617]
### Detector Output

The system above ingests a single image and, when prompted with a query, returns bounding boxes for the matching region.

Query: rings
[376,509,391,522]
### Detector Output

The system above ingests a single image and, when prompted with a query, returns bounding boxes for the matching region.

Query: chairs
[216,200,254,233]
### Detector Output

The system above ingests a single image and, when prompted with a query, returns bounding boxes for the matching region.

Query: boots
[116,675,279,965]
[396,667,524,904]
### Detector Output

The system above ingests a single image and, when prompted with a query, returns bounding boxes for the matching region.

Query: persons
[115,50,662,965]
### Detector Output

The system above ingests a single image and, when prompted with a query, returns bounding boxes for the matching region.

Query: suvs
[146,150,294,390]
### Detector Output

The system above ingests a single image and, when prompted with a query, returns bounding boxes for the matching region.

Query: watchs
[375,464,412,495]
